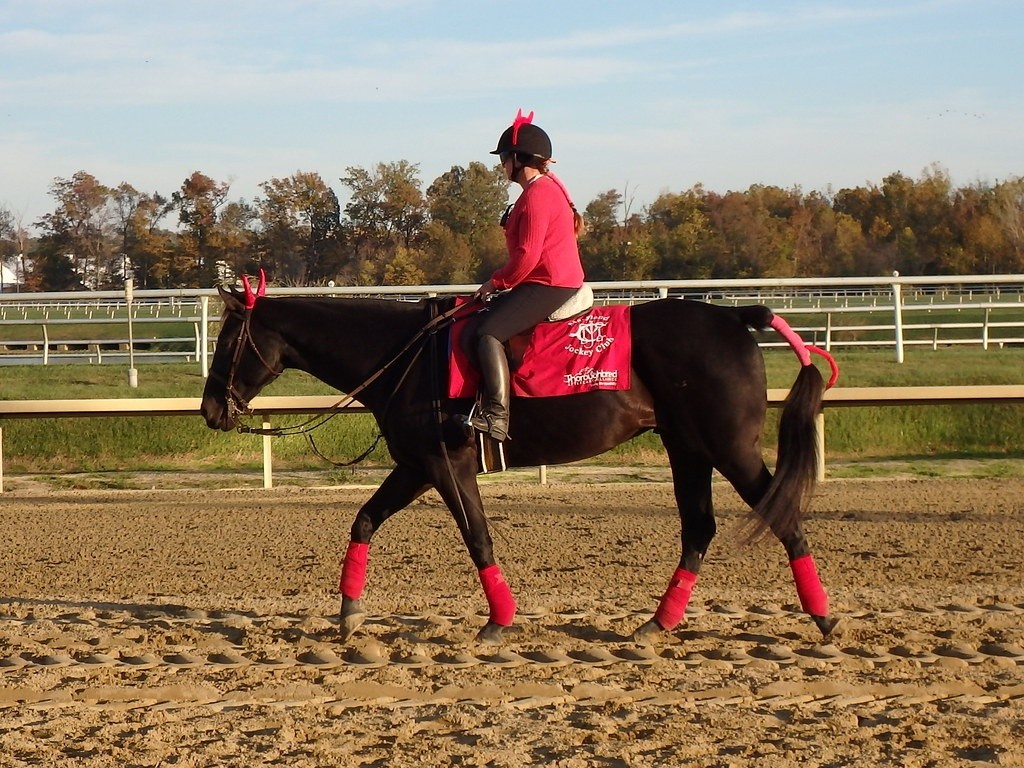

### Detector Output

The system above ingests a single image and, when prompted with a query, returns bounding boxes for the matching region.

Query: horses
[195,280,847,647]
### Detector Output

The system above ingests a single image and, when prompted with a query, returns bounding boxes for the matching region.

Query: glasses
[499,152,512,166]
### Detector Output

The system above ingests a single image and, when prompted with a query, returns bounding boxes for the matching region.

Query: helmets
[490,122,552,165]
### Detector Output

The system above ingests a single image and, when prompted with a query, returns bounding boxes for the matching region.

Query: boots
[453,334,510,442]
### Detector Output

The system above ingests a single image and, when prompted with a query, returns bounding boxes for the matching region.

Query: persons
[452,107,586,443]
